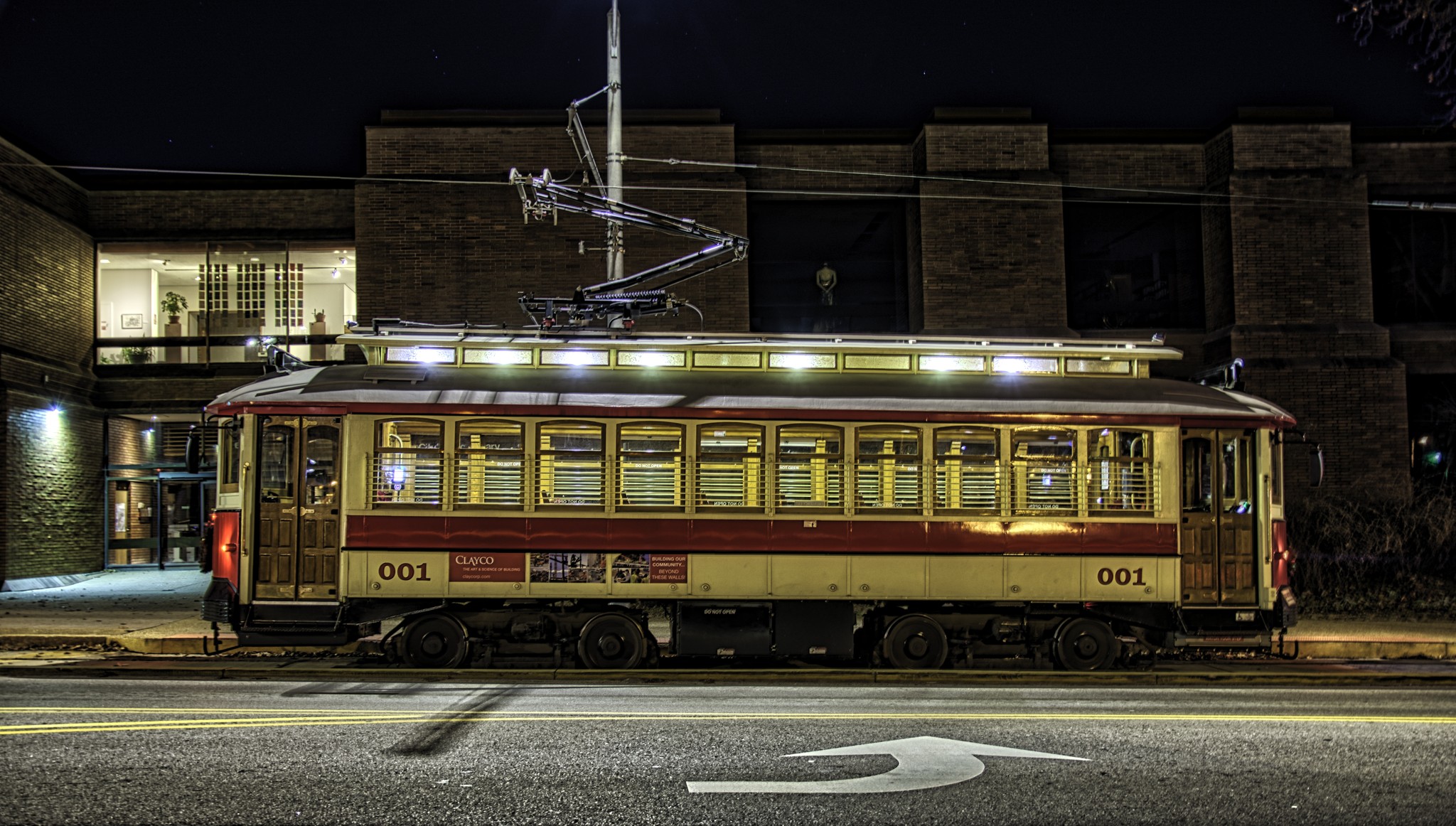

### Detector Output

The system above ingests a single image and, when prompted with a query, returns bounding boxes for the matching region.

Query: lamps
[333,257,348,278]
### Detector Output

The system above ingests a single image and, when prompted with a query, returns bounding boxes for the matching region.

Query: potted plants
[161,291,188,324]
[122,332,155,364]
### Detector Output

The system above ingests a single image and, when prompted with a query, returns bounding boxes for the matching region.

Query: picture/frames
[121,314,143,329]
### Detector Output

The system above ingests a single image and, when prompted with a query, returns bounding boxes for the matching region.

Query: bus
[187,82,1325,677]
